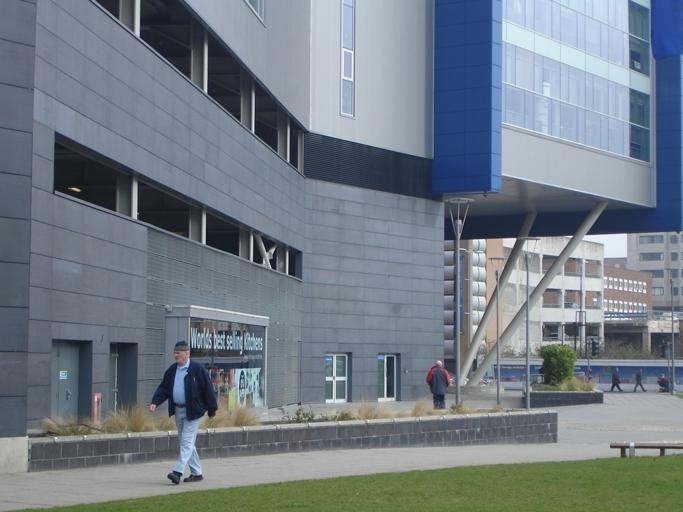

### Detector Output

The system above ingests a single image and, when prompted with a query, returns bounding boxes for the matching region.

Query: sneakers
[168,473,180,484]
[184,474,202,482]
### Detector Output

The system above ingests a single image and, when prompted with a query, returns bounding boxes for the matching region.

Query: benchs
[610,442,683,457]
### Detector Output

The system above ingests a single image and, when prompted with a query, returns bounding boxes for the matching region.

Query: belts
[174,402,185,407]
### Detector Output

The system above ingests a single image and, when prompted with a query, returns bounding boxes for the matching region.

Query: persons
[426,361,452,410]
[611,367,624,392]
[591,338,596,356]
[659,374,669,391]
[660,339,666,357]
[633,367,648,392]
[149,341,218,484]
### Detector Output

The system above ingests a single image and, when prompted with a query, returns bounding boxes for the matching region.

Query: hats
[175,340,189,351]
